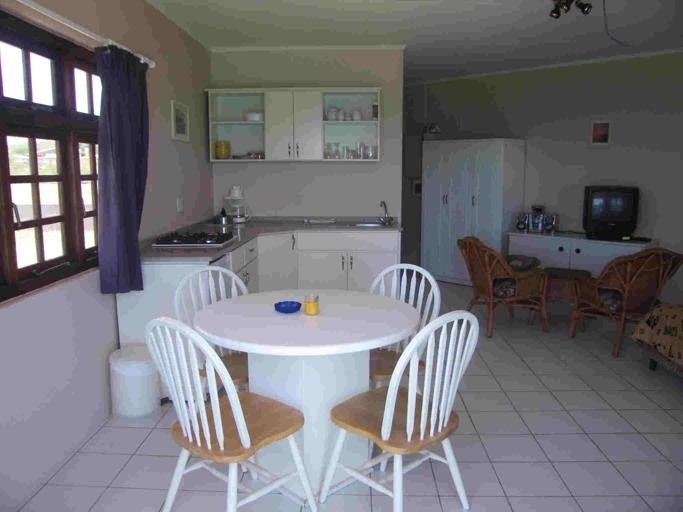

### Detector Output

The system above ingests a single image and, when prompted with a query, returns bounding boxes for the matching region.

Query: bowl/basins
[215,139,231,159]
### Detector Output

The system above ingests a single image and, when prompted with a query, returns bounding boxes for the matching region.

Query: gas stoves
[150,231,237,249]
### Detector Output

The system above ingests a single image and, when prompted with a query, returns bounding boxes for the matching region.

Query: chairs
[457,237,551,338]
[175,266,249,409]
[367,264,442,395]
[143,316,318,512]
[567,247,683,359]
[318,310,480,512]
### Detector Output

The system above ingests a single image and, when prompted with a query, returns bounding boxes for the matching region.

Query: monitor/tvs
[582,185,639,241]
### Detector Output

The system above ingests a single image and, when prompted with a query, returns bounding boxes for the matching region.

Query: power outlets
[176,197,184,214]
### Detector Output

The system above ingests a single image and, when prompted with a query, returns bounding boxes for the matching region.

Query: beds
[631,303,683,380]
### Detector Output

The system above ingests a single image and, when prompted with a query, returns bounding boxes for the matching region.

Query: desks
[527,267,592,333]
[192,288,420,503]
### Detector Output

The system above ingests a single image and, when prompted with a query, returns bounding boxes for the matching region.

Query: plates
[245,112,265,122]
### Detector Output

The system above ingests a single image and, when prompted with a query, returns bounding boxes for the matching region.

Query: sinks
[349,223,384,227]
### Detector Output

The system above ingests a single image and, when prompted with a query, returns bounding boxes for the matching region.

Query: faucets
[377,201,393,227]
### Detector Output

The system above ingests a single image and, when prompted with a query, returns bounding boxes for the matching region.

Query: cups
[232,203,249,223]
[326,142,378,161]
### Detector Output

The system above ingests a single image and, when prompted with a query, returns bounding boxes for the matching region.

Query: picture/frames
[585,117,616,150]
[170,98,190,143]
[413,179,421,196]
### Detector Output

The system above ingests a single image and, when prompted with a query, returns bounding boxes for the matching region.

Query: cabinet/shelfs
[299,219,400,298]
[506,231,662,277]
[421,138,527,288]
[116,216,258,402]
[204,86,385,163]
[258,216,298,291]
